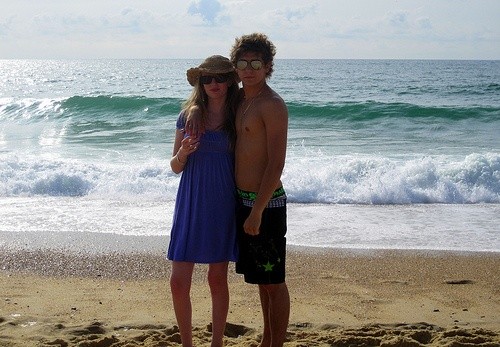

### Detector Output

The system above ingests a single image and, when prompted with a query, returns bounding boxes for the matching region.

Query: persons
[167,56,239,347]
[184,33,290,347]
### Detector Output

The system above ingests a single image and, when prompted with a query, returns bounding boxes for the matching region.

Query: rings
[194,147,196,150]
[190,144,192,147]
[189,125,192,128]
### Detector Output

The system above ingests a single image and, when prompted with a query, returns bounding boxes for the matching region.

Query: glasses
[237,59,268,70]
[198,74,229,84]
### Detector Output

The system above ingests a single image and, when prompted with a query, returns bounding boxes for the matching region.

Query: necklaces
[243,83,267,115]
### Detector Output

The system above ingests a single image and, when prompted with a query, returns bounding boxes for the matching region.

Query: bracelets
[175,152,185,166]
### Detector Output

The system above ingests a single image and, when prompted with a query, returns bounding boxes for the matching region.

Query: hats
[186,55,241,86]
